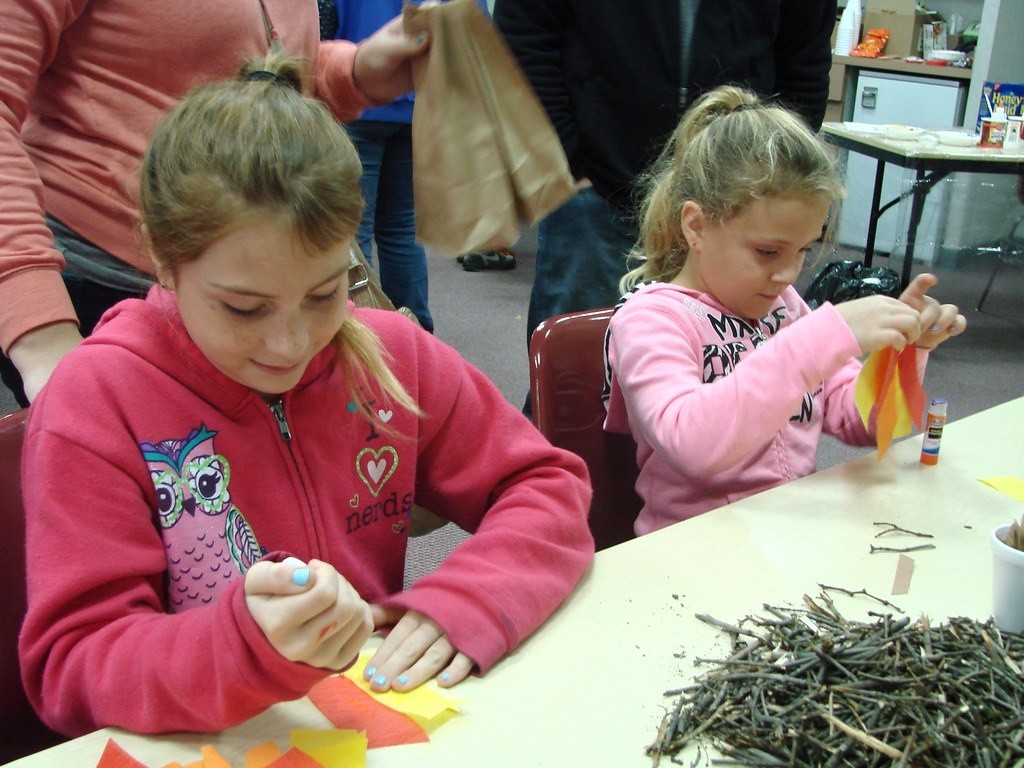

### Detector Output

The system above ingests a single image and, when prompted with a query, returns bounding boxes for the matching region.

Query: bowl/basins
[937,131,978,147]
[883,124,925,140]
[933,49,966,60]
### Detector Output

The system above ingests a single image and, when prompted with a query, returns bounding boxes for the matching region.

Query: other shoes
[456,247,518,271]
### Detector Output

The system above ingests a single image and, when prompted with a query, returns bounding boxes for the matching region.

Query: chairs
[532,306,643,556]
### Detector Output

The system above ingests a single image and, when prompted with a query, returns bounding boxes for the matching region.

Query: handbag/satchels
[358,306,452,538]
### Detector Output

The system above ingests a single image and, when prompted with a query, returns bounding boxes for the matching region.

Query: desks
[821,120,1024,308]
[0,401,1024,768]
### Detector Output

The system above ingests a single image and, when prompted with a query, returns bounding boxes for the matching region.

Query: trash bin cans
[804,260,901,311]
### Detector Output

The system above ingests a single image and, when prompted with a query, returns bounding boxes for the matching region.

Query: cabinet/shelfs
[817,63,857,165]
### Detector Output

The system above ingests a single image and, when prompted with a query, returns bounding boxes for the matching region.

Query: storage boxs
[923,23,947,60]
[862,0,933,61]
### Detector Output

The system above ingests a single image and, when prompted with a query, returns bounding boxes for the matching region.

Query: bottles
[991,100,1007,121]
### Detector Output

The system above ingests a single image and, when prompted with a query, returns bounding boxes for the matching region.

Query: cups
[1007,116,1024,145]
[834,0,861,57]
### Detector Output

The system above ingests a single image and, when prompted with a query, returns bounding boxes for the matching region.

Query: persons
[16,49,596,740]
[0,0,838,424]
[601,84,967,538]
